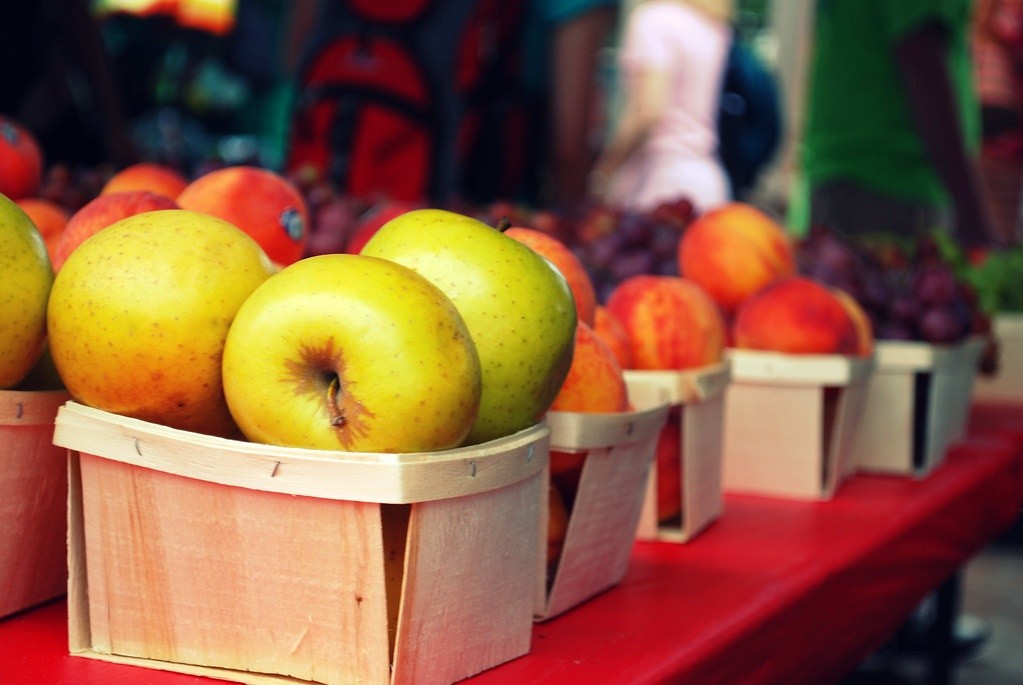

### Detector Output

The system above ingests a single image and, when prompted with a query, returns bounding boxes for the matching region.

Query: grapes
[30,158,1002,375]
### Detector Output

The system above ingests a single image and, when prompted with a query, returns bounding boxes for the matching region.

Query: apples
[0,194,579,665]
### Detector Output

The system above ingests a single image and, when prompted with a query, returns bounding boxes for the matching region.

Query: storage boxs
[714,349,879,501]
[0,386,75,622]
[852,339,980,473]
[524,403,678,623]
[616,351,740,544]
[51,401,558,685]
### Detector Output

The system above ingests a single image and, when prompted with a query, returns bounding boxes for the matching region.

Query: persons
[803,0,994,663]
[278,0,785,215]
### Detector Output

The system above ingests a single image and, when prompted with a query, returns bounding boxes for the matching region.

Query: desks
[0,398,1023,685]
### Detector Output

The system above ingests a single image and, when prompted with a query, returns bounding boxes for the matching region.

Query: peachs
[0,110,871,574]
